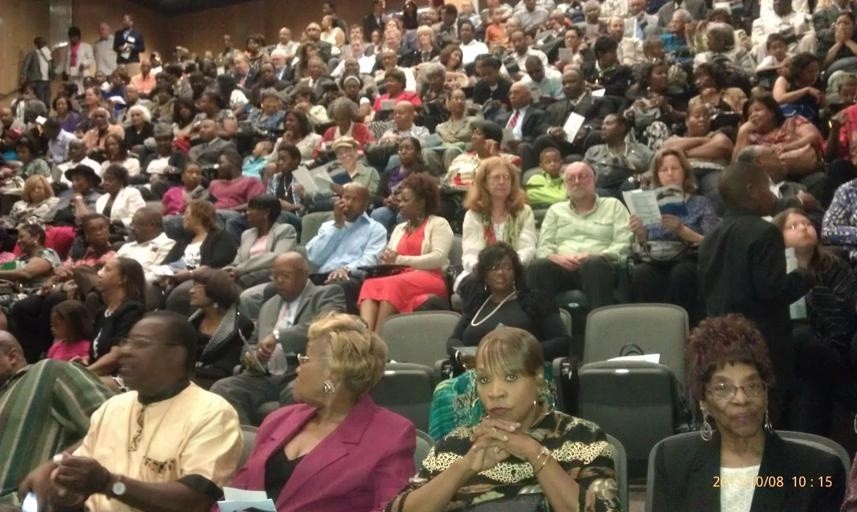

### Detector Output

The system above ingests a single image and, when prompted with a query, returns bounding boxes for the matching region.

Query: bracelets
[530,446,550,478]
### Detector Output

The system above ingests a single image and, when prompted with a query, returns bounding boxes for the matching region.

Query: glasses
[190,284,207,291]
[489,264,515,271]
[785,220,813,231]
[489,174,512,183]
[565,173,592,184]
[120,336,179,351]
[297,353,335,365]
[706,381,766,400]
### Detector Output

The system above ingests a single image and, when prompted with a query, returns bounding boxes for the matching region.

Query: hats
[332,136,360,151]
[64,164,101,186]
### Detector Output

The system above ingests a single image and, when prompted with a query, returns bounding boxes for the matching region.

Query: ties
[508,111,520,130]
[265,301,290,377]
[311,79,317,89]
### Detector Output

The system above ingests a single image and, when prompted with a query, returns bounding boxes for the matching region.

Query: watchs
[106,473,127,500]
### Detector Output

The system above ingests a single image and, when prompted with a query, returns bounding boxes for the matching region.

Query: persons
[210,309,416,512]
[1,330,113,511]
[649,314,842,512]
[1,0,857,430]
[20,313,243,512]
[381,327,620,512]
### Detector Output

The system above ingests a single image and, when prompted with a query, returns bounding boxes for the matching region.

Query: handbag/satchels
[780,144,817,175]
